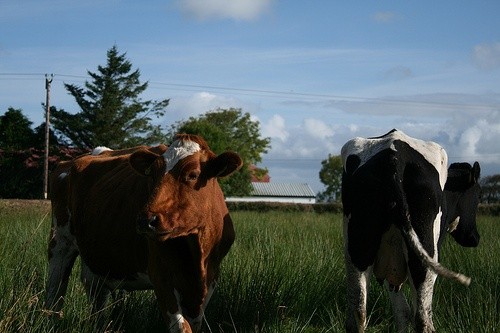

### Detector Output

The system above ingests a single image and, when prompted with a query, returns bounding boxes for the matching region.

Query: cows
[341,128,482,333]
[41,131,242,333]
